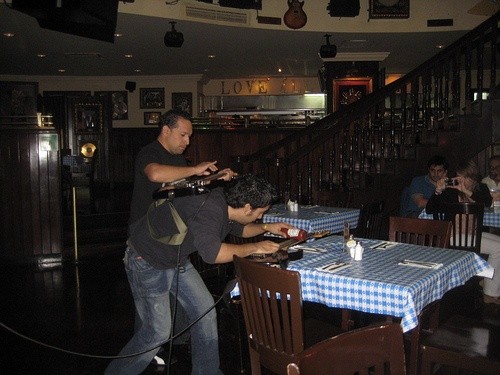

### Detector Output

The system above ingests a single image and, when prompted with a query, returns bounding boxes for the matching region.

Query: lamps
[320,34,337,58]
[164,21,185,47]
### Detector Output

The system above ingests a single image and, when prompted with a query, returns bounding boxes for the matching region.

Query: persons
[102,173,295,375]
[127,111,235,366]
[399,155,500,238]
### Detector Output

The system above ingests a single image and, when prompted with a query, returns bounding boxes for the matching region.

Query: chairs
[190,190,500,375]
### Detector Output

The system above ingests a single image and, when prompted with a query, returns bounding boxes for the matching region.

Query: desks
[262,203,361,244]
[228,234,495,375]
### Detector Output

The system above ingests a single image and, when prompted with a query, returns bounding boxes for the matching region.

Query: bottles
[280,227,313,241]
[290,199,299,212]
[349,233,365,261]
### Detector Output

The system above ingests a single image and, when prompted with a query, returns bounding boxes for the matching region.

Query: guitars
[152,169,240,201]
[245,230,331,270]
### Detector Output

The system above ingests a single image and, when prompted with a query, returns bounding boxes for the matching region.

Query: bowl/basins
[288,248,303,261]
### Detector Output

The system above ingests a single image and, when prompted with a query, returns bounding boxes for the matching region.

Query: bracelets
[262,224,269,231]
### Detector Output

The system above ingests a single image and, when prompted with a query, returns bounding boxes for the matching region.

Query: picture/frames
[369,0,410,19]
[144,112,162,126]
[73,102,104,135]
[172,91,193,120]
[333,76,372,114]
[140,88,165,109]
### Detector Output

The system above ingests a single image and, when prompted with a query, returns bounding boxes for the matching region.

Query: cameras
[447,179,456,186]
